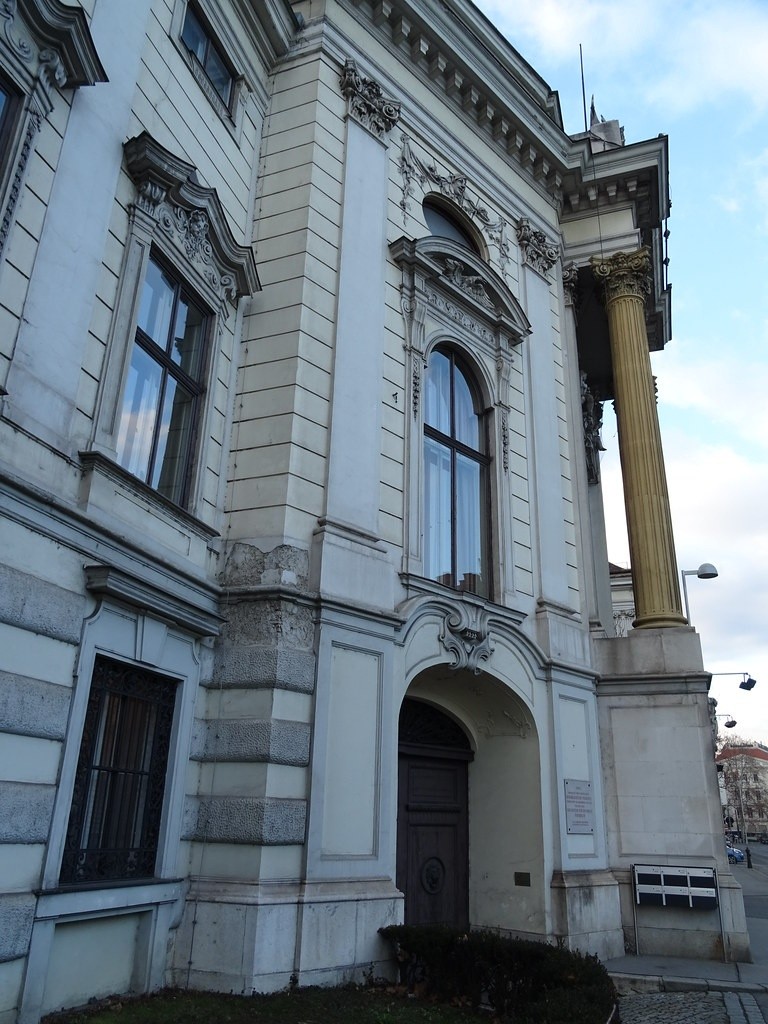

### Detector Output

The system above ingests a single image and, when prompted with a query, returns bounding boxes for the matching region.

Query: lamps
[681,563,718,624]
[717,764,724,772]
[715,714,737,728]
[712,670,755,690]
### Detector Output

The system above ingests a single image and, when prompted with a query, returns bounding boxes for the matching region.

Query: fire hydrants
[745,847,752,869]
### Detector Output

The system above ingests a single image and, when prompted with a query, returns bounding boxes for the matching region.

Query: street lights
[681,564,717,625]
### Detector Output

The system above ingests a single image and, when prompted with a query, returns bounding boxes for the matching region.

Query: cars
[726,845,744,864]
